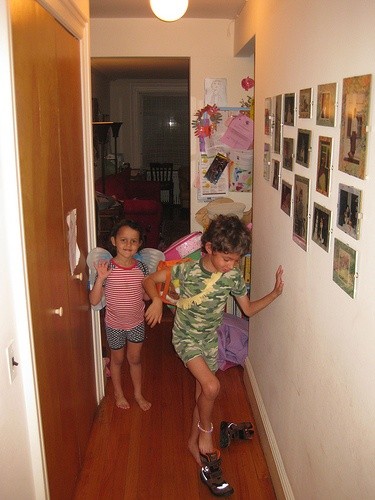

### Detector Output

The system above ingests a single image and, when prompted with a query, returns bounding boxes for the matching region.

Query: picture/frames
[315,81,338,129]
[298,87,314,119]
[270,158,281,190]
[308,201,334,254]
[281,136,294,172]
[336,184,365,240]
[314,135,334,198]
[272,93,281,155]
[290,173,312,254]
[294,127,313,169]
[332,238,359,300]
[279,178,293,217]
[283,92,297,127]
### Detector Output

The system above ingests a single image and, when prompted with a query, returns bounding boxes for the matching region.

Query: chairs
[149,162,175,208]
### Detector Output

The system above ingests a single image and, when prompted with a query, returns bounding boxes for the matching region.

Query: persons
[88,222,153,411]
[142,214,283,466]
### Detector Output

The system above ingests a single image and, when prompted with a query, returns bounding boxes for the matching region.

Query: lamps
[150,0,190,23]
[111,120,123,174]
[93,121,115,194]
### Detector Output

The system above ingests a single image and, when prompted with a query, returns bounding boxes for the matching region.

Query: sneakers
[199,453,233,496]
[220,421,255,448]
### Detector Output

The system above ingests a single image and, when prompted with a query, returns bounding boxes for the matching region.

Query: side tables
[130,167,147,181]
[98,200,124,239]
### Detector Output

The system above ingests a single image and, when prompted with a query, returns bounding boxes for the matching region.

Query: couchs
[100,165,163,249]
[178,165,190,209]
